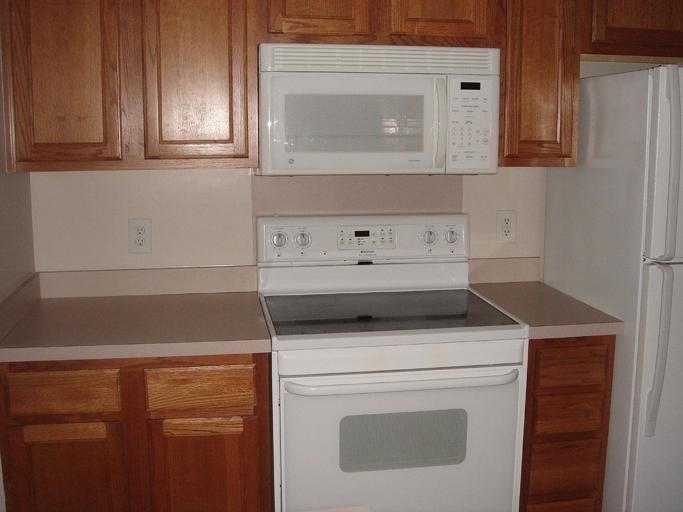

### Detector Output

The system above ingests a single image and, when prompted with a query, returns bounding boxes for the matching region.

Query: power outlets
[495,209,519,245]
[128,217,154,254]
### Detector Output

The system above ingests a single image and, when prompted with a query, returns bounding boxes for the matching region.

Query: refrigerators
[542,62,682,511]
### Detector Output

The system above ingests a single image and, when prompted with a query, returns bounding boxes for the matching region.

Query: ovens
[267,340,530,512]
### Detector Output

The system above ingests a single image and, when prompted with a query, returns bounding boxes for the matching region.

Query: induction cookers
[255,288,532,350]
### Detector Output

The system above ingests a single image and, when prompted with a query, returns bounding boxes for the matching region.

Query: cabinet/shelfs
[257,1,502,49]
[501,0,581,168]
[2,342,273,512]
[579,0,683,65]
[521,321,620,512]
[2,0,257,173]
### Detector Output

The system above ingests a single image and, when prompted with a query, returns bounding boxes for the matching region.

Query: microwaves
[253,40,500,175]
